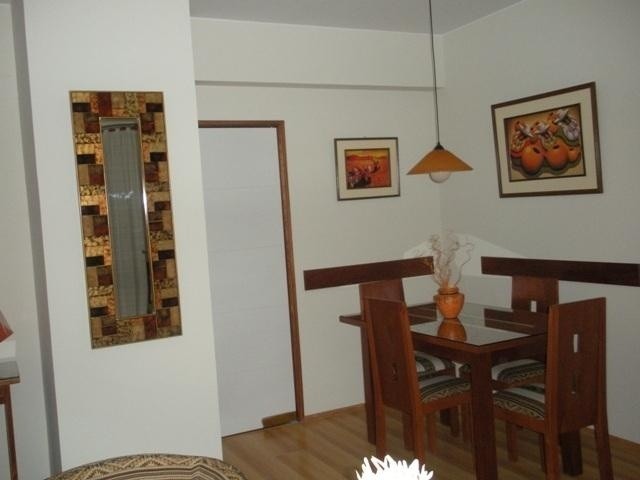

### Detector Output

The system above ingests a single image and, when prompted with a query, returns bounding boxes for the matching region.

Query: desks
[336,298,586,479]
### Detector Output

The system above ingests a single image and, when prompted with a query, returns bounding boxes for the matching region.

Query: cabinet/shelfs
[0,359,21,479]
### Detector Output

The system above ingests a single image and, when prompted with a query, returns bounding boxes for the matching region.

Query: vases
[431,288,467,321]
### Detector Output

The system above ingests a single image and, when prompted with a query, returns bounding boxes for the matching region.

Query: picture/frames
[488,79,605,200]
[332,135,403,201]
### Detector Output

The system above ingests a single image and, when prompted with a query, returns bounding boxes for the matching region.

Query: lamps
[405,1,476,186]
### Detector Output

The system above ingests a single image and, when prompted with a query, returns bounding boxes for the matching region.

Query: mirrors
[69,88,182,351]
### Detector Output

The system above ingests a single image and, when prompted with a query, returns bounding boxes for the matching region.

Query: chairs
[459,272,562,463]
[360,295,474,467]
[356,275,409,308]
[491,295,619,479]
